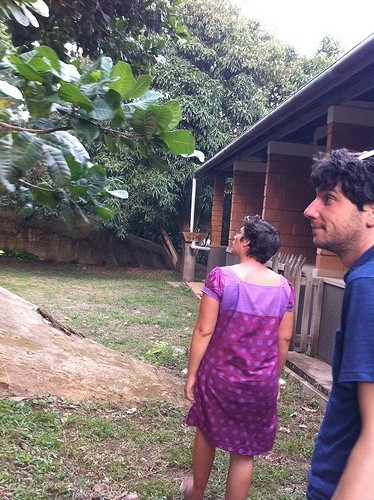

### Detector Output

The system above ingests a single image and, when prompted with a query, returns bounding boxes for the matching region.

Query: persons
[303,148,373,500]
[180,213,294,500]
